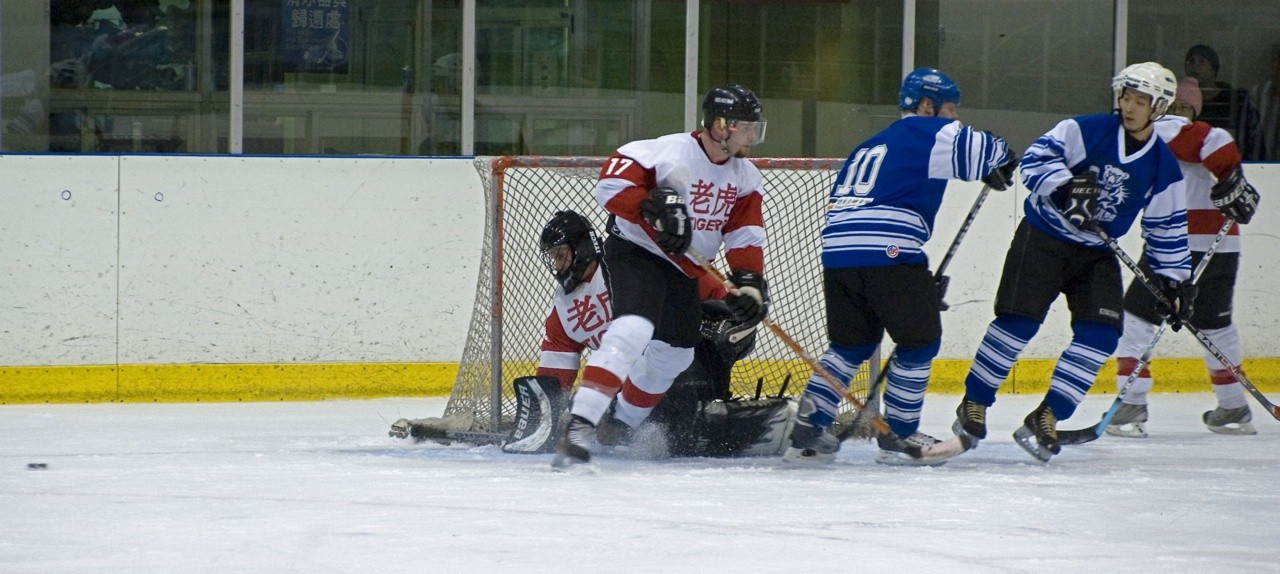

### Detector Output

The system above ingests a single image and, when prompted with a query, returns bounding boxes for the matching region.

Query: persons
[1166,45,1268,160]
[1103,116,1260,437]
[550,83,770,471]
[952,62,1200,462]
[503,209,799,457]
[784,68,1018,469]
[424,50,527,156]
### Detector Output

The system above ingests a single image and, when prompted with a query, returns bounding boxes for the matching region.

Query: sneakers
[1203,405,1258,436]
[951,394,987,449]
[1013,401,1061,463]
[550,409,593,469]
[1103,402,1151,437]
[789,418,841,454]
[874,431,945,464]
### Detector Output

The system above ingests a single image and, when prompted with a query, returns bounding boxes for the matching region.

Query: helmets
[898,66,961,117]
[1111,62,1176,122]
[539,210,603,295]
[701,85,762,128]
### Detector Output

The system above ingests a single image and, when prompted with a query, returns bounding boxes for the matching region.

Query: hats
[1177,75,1203,118]
[1184,45,1221,73]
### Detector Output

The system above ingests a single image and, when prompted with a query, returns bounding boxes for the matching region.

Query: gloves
[1060,172,1098,230]
[1155,283,1200,332]
[640,186,692,259]
[982,149,1019,192]
[726,270,768,321]
[1211,168,1260,225]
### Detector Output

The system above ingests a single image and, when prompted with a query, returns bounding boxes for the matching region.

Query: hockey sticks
[685,245,973,460]
[1087,221,1280,422]
[410,422,507,443]
[1055,215,1235,445]
[803,147,1017,457]
